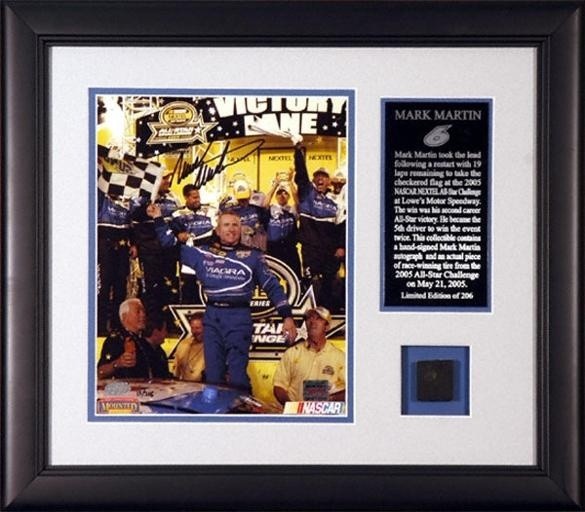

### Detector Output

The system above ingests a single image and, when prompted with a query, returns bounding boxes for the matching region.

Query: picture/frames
[2,3,583,508]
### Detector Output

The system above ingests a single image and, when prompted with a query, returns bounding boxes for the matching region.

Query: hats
[306,306,332,327]
[227,166,346,200]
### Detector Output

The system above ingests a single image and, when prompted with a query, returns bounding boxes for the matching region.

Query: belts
[207,301,250,309]
